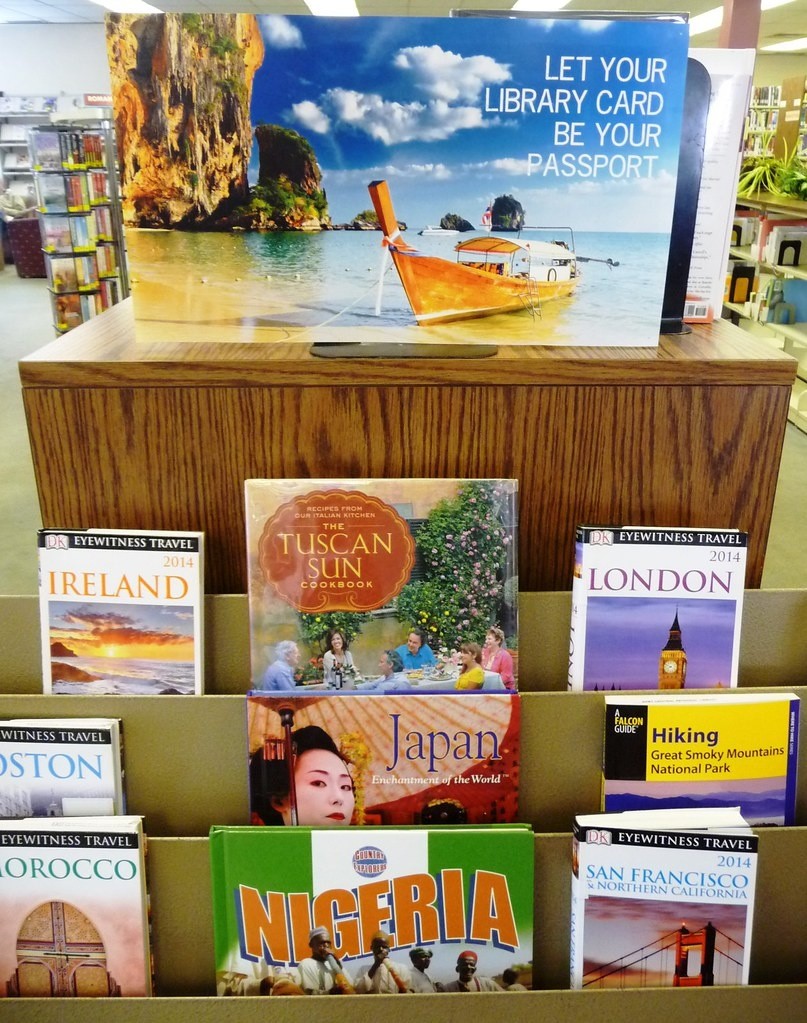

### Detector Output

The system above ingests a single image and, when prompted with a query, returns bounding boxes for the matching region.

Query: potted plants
[737,132,785,199]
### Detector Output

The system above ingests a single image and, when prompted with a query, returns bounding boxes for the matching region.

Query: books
[599,693,800,827]
[27,126,122,336]
[208,823,535,998]
[247,689,521,823]
[566,523,748,692]
[570,805,758,991]
[37,528,206,696]
[243,476,518,693]
[0,717,126,818]
[722,84,807,324]
[0,814,156,998]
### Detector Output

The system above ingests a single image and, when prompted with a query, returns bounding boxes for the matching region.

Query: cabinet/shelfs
[721,190,807,437]
[0,112,130,336]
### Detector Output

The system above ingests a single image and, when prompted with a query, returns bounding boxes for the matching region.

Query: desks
[16,300,799,592]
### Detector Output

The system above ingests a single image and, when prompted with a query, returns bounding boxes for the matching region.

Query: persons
[434,951,528,992]
[346,649,412,690]
[395,628,443,670]
[454,643,485,691]
[408,946,439,993]
[297,926,353,995]
[249,725,354,826]
[260,640,300,691]
[480,626,516,690]
[355,930,411,995]
[322,629,359,684]
